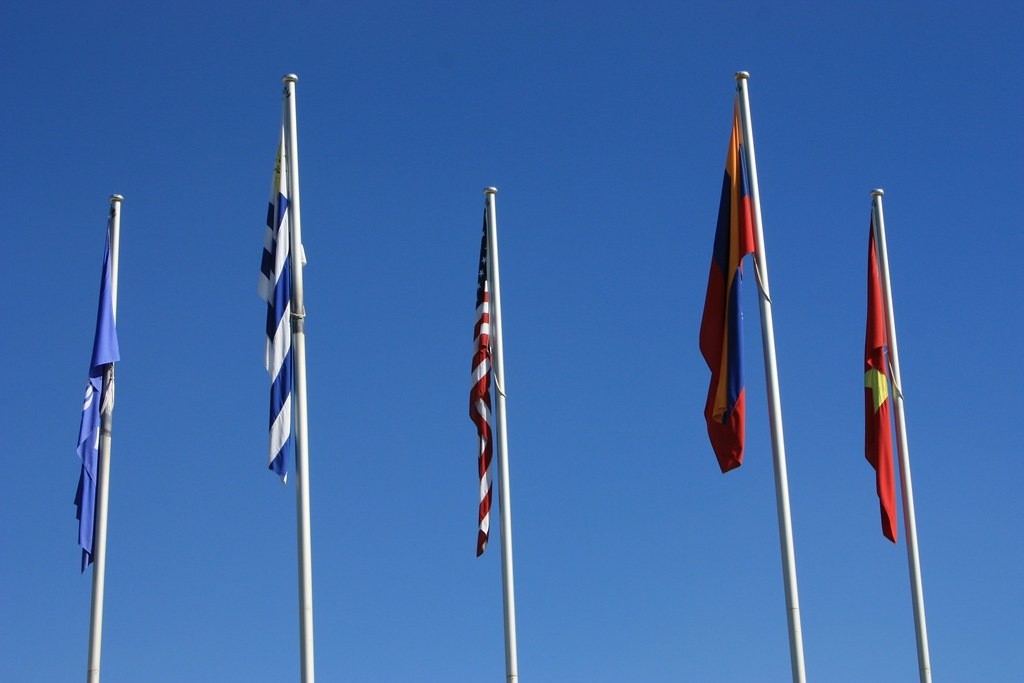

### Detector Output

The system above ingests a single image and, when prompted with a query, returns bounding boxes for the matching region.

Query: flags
[258,97,302,483]
[698,95,758,473]
[73,216,121,574]
[468,201,495,558]
[864,210,898,544]
